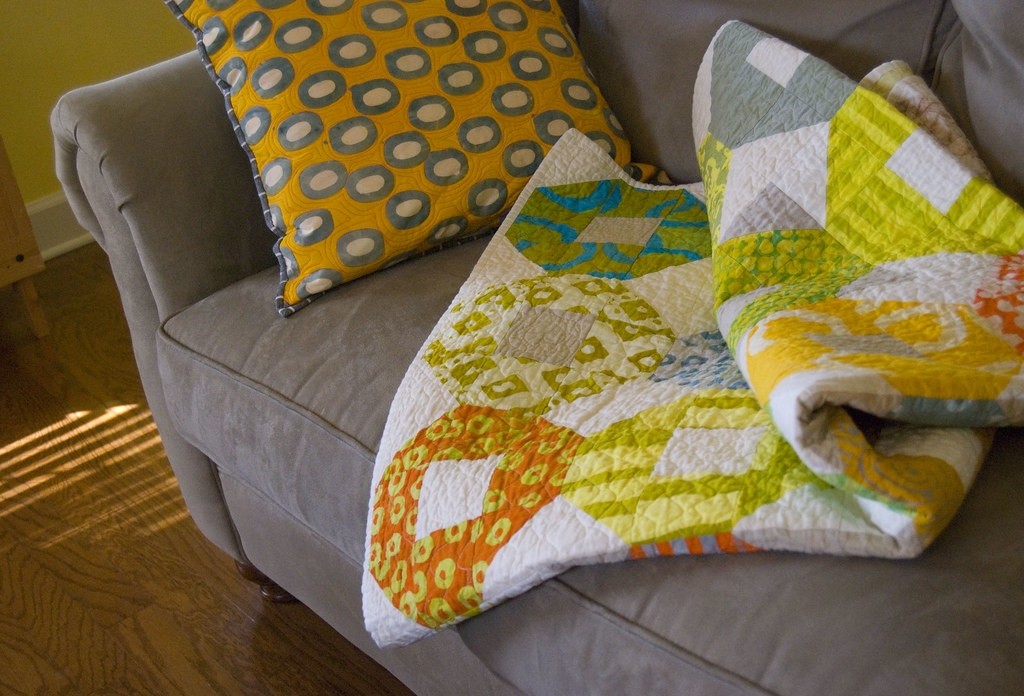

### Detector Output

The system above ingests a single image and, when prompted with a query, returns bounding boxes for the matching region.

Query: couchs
[51,47,1023,695]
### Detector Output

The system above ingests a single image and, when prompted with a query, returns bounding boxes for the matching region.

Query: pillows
[551,0,944,184]
[162,0,677,321]
[928,1,1024,207]
